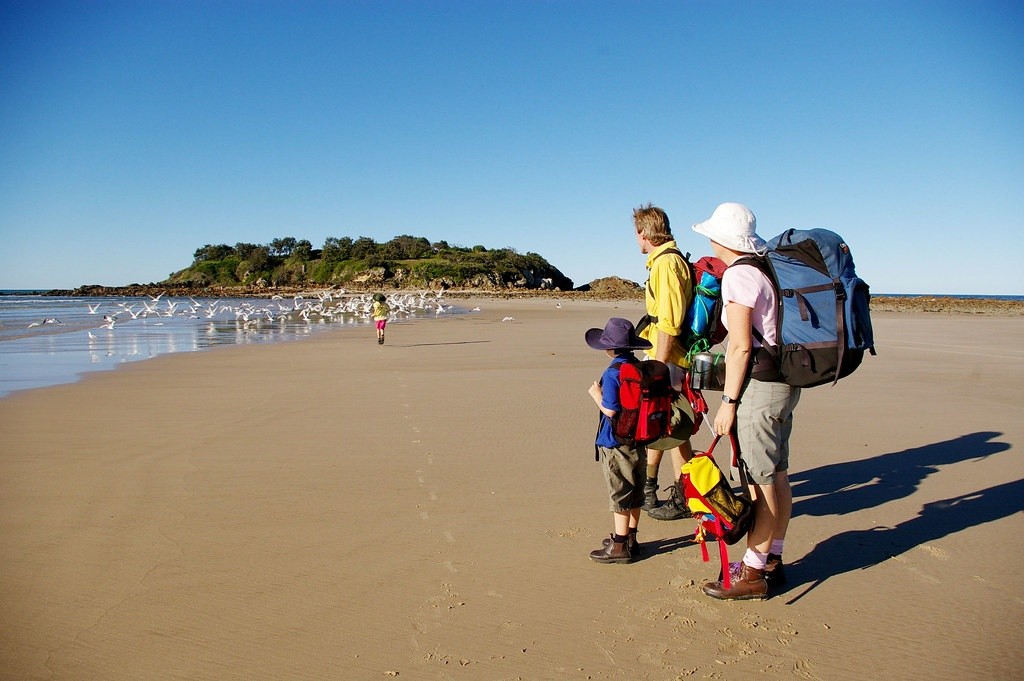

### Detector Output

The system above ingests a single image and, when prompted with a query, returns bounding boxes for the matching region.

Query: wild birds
[556,302,562,309]
[86,303,102,314]
[100,286,457,330]
[87,331,100,342]
[502,316,515,322]
[27,317,62,328]
[542,277,552,284]
[473,306,481,312]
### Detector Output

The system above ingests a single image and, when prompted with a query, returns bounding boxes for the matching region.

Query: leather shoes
[588,533,631,564]
[602,531,639,553]
[733,553,785,585]
[702,560,768,600]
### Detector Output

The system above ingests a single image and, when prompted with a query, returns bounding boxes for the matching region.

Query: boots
[648,478,692,521]
[639,476,660,511]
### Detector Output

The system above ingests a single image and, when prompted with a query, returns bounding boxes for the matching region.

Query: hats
[692,202,768,253]
[373,293,386,302]
[585,318,653,351]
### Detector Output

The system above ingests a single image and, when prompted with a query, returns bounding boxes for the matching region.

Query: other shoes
[379,335,384,344]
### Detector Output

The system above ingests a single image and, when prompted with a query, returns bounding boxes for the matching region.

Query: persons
[587,201,802,602]
[372,292,391,345]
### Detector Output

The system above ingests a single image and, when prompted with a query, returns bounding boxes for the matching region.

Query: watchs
[721,394,738,404]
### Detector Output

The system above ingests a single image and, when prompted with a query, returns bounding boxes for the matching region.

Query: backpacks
[598,357,672,447]
[648,247,728,351]
[724,228,877,388]
[680,431,754,546]
